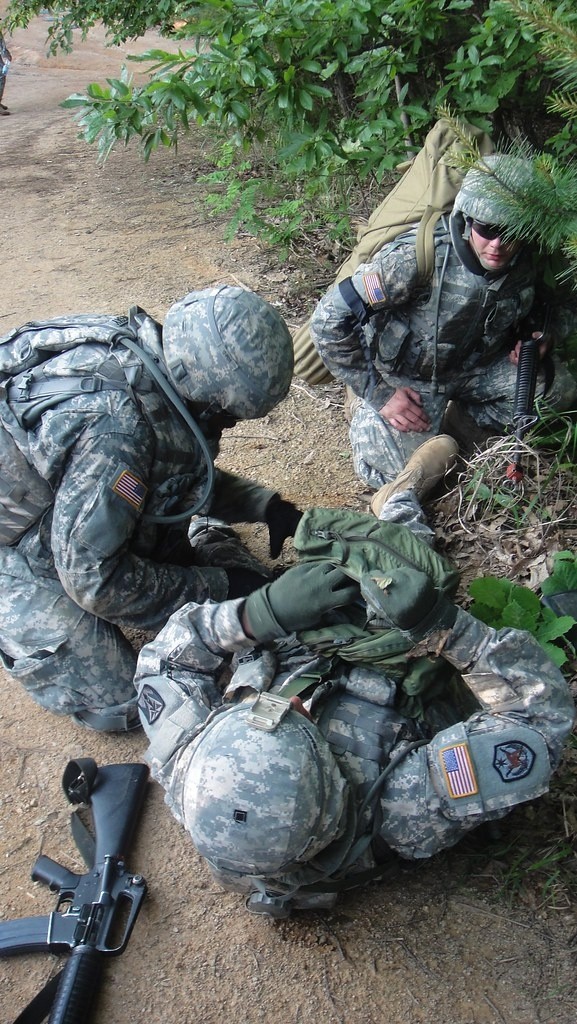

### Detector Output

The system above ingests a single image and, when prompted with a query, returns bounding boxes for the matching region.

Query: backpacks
[261,506,463,719]
[293,119,506,387]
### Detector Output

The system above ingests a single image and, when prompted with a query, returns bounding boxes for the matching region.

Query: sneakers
[444,399,504,453]
[371,434,460,517]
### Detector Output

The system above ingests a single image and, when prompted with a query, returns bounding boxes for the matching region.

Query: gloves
[264,500,304,559]
[359,566,457,648]
[226,568,264,601]
[248,562,359,640]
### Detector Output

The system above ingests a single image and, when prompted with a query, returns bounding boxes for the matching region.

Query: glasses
[471,220,516,243]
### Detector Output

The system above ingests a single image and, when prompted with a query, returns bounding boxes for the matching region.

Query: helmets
[451,155,540,272]
[164,692,350,877]
[161,285,294,425]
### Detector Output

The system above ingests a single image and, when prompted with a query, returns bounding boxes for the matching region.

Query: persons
[0,17,13,115]
[306,150,575,491]
[131,434,577,924]
[1,284,307,737]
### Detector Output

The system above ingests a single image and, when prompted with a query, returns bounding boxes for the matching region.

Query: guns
[1,756,153,1024]
